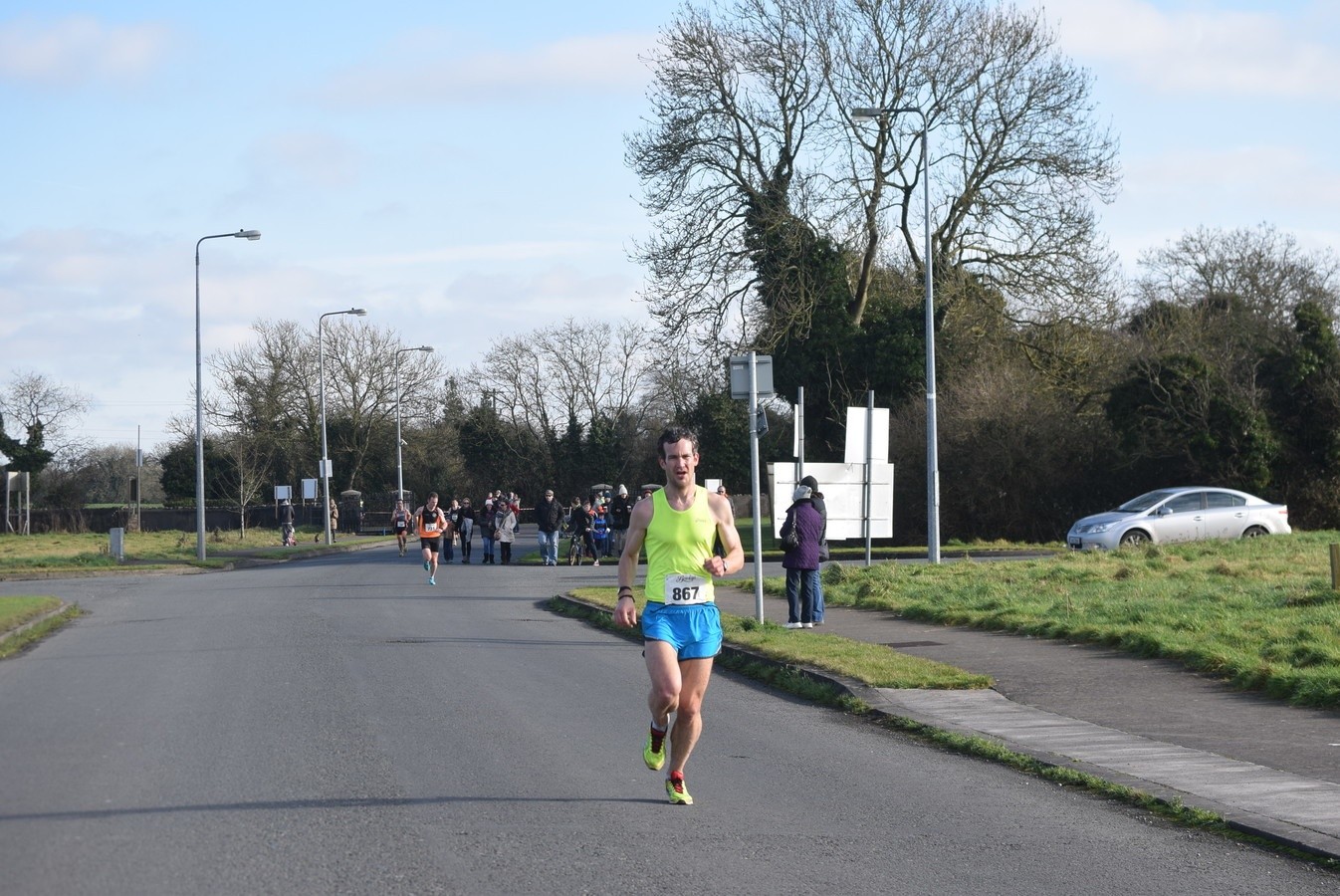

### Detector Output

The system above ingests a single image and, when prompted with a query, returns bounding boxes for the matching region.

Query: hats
[485,499,494,505]
[792,485,812,501]
[604,491,611,498]
[546,490,554,495]
[800,475,818,494]
[618,484,628,495]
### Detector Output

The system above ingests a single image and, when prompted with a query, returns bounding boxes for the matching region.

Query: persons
[799,475,826,626]
[442,497,475,564]
[713,485,736,558]
[614,429,745,806]
[329,499,338,543]
[495,499,516,566]
[280,499,295,547]
[780,485,823,629]
[390,498,412,556]
[413,491,448,585]
[478,499,498,565]
[562,484,653,566]
[534,489,565,567]
[487,489,521,534]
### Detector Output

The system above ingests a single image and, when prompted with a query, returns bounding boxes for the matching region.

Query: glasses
[500,504,507,506]
[545,495,552,497]
[463,502,470,504]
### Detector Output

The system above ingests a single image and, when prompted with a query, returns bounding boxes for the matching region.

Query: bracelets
[617,585,632,595]
[408,519,410,521]
[618,593,635,603]
[440,526,444,530]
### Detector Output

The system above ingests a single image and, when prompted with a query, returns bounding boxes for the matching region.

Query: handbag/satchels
[818,543,829,562]
[781,509,799,552]
[494,528,500,541]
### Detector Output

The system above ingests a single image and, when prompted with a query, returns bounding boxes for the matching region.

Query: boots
[483,553,495,563]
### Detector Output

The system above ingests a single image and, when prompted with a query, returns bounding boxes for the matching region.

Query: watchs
[723,560,727,573]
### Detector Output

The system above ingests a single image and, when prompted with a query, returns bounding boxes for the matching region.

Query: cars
[1066,484,1293,554]
[558,514,578,539]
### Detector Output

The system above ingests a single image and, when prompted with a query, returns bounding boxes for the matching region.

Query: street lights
[853,106,944,563]
[317,308,368,548]
[196,228,263,564]
[394,346,437,509]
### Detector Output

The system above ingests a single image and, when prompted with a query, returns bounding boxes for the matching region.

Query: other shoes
[502,557,510,565]
[403,542,408,552]
[424,560,430,571]
[461,555,470,562]
[430,578,435,585]
[812,620,825,627]
[445,559,453,563]
[399,549,403,557]
[543,560,556,566]
[593,560,600,566]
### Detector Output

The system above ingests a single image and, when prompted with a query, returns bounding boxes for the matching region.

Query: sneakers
[642,722,669,771]
[782,621,803,629]
[801,621,813,629]
[665,769,693,806]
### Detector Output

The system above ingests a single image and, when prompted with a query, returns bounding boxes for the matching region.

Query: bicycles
[564,526,588,566]
